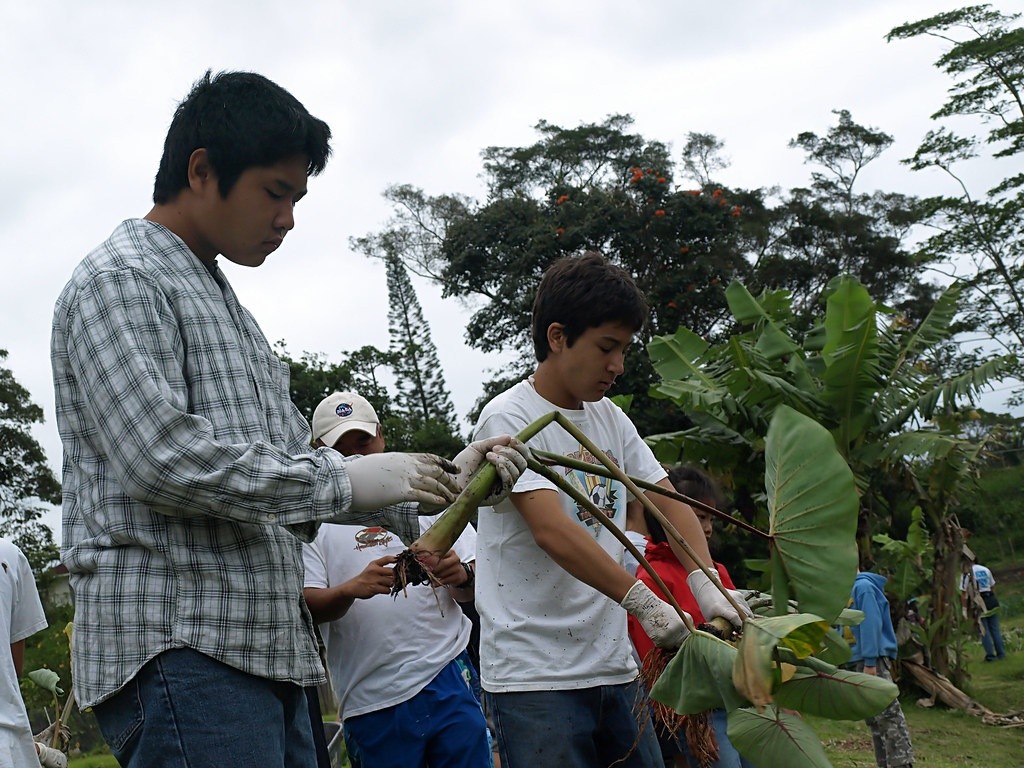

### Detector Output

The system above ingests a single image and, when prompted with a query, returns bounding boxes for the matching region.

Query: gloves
[685,567,754,634]
[421,432,529,512]
[340,452,463,511]
[34,742,67,768]
[620,580,694,650]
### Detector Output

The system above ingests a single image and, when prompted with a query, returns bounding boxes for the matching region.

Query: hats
[312,392,379,448]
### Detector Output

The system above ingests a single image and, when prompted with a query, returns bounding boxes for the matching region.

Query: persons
[302,391,493,768]
[834,566,916,768]
[50,70,532,768]
[624,466,803,768]
[33,720,71,768]
[621,495,685,768]
[0,538,48,768]
[474,251,754,768]
[959,557,1006,662]
